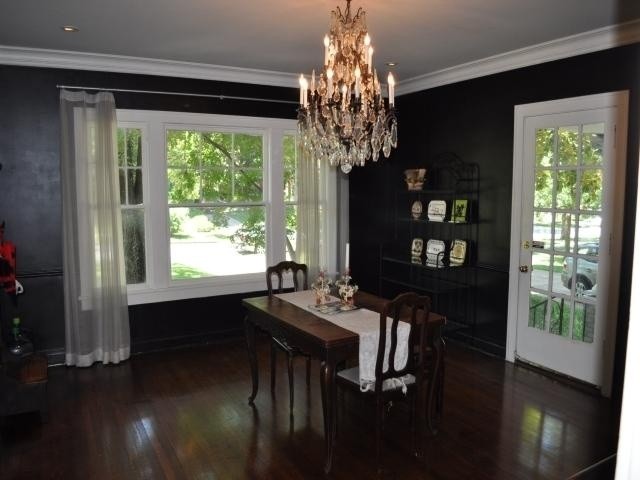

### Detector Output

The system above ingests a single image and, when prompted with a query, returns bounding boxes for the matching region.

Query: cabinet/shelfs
[378,162,480,362]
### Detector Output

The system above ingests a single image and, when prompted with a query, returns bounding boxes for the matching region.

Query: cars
[561,242,599,297]
[234,224,297,254]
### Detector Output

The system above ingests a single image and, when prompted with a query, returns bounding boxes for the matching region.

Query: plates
[410,200,467,264]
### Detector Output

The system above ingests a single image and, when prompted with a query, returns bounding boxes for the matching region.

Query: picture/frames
[449,240,469,265]
[409,237,423,258]
[450,200,469,222]
[427,199,448,223]
[424,240,446,267]
[410,201,424,218]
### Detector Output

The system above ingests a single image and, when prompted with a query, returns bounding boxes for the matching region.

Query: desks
[0,350,48,424]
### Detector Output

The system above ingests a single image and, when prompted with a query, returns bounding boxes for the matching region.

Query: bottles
[9,318,24,355]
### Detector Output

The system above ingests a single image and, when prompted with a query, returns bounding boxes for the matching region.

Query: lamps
[294,0,402,175]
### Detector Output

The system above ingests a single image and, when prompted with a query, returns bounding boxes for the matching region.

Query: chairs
[337,290,431,472]
[266,262,313,397]
